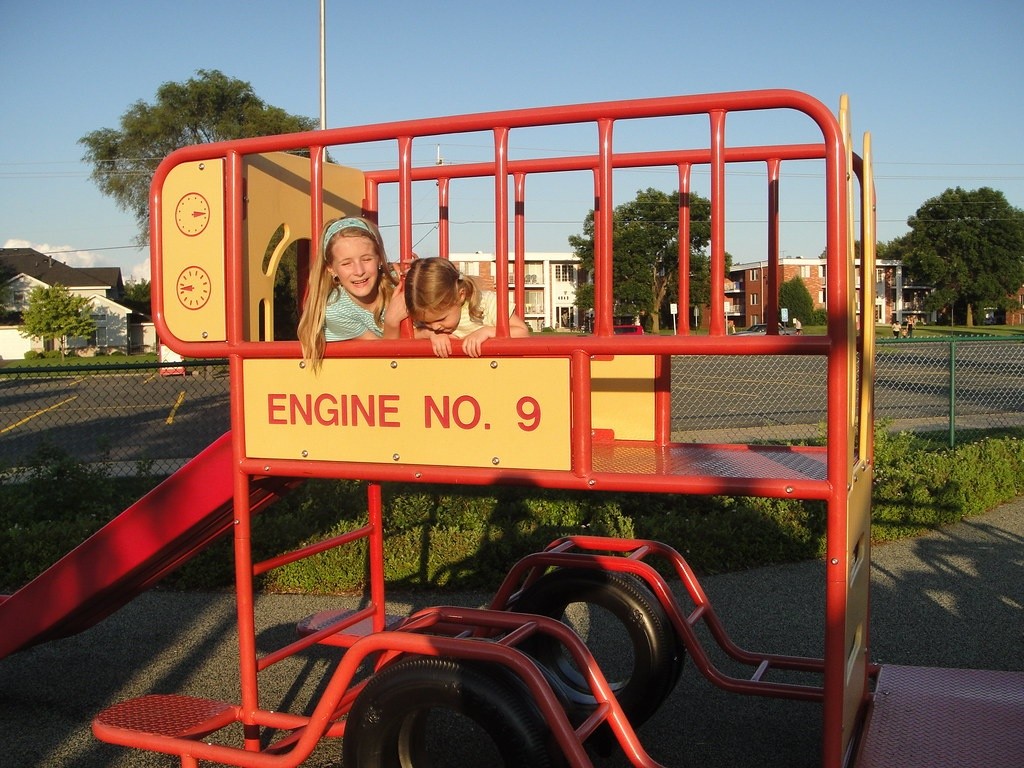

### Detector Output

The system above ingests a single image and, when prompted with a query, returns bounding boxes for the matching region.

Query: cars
[730,322,796,336]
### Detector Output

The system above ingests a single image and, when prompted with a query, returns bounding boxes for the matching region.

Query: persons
[571,314,594,332]
[795,319,802,336]
[902,316,914,339]
[297,214,529,358]
[892,320,901,340]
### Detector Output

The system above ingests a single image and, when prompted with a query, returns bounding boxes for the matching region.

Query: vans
[613,324,644,335]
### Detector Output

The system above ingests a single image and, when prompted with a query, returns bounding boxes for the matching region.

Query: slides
[0,429,302,668]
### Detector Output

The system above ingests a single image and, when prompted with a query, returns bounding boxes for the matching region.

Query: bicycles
[794,328,804,336]
